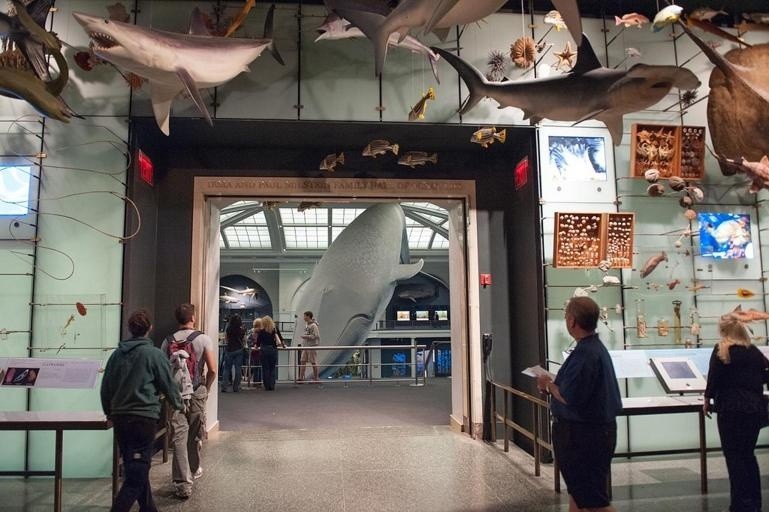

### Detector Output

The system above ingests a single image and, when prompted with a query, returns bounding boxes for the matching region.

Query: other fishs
[468,125,509,146]
[541,11,566,30]
[615,10,649,31]
[395,148,440,169]
[239,287,257,298]
[359,138,401,160]
[406,86,434,121]
[721,304,769,335]
[689,6,726,24]
[332,0,587,78]
[649,2,688,31]
[299,10,466,83]
[318,149,348,171]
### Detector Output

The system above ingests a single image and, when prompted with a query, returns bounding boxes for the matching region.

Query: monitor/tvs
[396,308,449,324]
[0,155,42,239]
[539,127,616,204]
[698,211,753,260]
[649,356,707,394]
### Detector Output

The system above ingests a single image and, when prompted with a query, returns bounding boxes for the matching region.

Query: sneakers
[191,466,203,480]
[176,486,192,498]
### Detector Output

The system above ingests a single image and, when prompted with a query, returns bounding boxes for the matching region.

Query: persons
[159,303,216,501]
[221,311,321,392]
[701,313,769,512]
[100,308,191,512]
[536,294,623,512]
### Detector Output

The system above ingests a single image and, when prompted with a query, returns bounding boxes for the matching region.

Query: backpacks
[165,330,204,391]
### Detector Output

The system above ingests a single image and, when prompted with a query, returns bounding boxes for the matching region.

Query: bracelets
[546,380,552,394]
